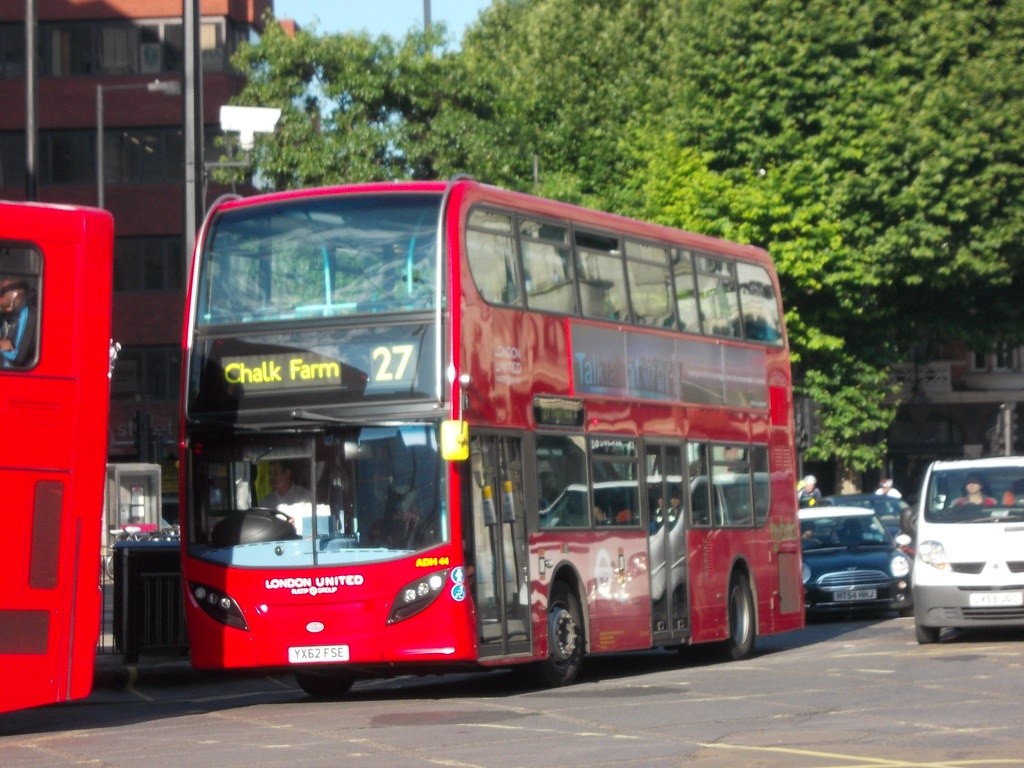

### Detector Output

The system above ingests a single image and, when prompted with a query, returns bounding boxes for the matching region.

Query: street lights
[1001,402,1015,457]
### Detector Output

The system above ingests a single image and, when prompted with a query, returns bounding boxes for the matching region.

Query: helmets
[803,475,817,484]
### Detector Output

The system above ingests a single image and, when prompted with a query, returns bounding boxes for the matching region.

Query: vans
[900,456,1024,643]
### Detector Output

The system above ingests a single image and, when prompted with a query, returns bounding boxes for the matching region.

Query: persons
[797,475,822,508]
[259,461,312,535]
[874,478,902,499]
[538,458,681,525]
[0,277,37,369]
[948,473,999,524]
[801,522,816,548]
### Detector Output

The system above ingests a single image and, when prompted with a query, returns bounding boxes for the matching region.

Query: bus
[0,205,117,728]
[172,172,806,702]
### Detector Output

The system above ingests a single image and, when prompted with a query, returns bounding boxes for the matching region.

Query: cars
[797,505,913,622]
[815,494,910,538]
